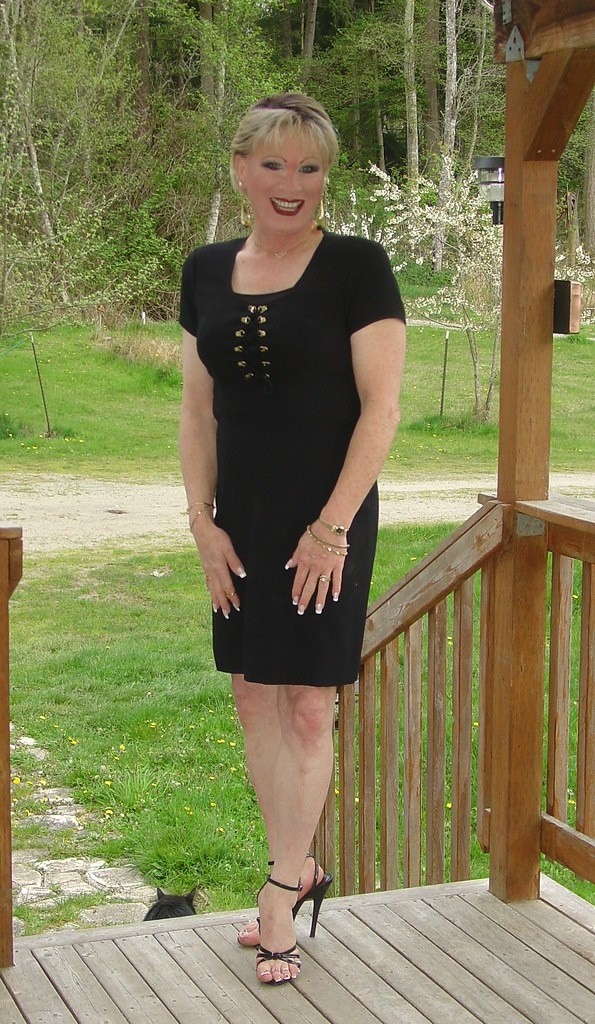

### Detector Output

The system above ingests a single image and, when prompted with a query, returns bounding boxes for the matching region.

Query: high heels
[237,851,333,948]
[255,876,302,986]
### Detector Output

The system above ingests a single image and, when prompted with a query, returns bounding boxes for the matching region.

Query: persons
[176,92,407,985]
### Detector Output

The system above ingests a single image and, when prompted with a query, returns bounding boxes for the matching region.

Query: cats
[143,886,197,921]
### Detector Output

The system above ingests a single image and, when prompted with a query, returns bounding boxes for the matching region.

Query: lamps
[471,156,504,228]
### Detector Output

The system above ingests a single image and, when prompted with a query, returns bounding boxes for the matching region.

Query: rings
[226,592,235,599]
[206,576,211,581]
[320,576,331,582]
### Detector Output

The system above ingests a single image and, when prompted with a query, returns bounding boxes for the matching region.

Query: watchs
[319,517,349,535]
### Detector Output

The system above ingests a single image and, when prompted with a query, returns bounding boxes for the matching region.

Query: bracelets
[186,501,215,532]
[307,525,351,556]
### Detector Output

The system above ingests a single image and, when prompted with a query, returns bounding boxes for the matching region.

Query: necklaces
[253,240,310,259]
